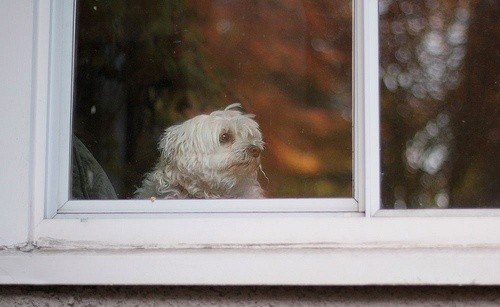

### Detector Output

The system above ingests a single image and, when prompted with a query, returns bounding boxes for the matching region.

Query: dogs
[133,101,271,198]
